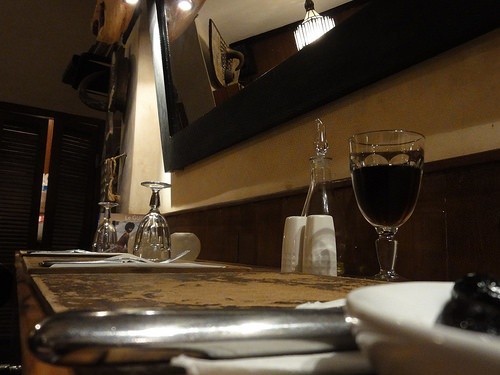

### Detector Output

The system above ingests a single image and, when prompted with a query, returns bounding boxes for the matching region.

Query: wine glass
[132,182,170,263]
[348,129,426,281]
[92,202,120,254]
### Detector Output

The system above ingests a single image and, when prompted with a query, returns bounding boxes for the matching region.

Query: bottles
[301,117,345,275]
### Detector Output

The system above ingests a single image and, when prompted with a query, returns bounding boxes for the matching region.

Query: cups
[302,215,337,276]
[280,216,307,273]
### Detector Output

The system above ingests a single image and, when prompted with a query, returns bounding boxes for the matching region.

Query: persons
[115,222,135,253]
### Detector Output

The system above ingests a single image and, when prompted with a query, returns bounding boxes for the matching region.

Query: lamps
[294,0,336,51]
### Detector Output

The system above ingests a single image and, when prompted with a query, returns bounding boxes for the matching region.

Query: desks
[16,248,395,375]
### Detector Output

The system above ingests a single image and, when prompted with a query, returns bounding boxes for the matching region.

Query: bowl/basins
[346,281,500,374]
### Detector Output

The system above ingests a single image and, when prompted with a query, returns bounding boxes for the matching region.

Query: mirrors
[155,0,499,172]
